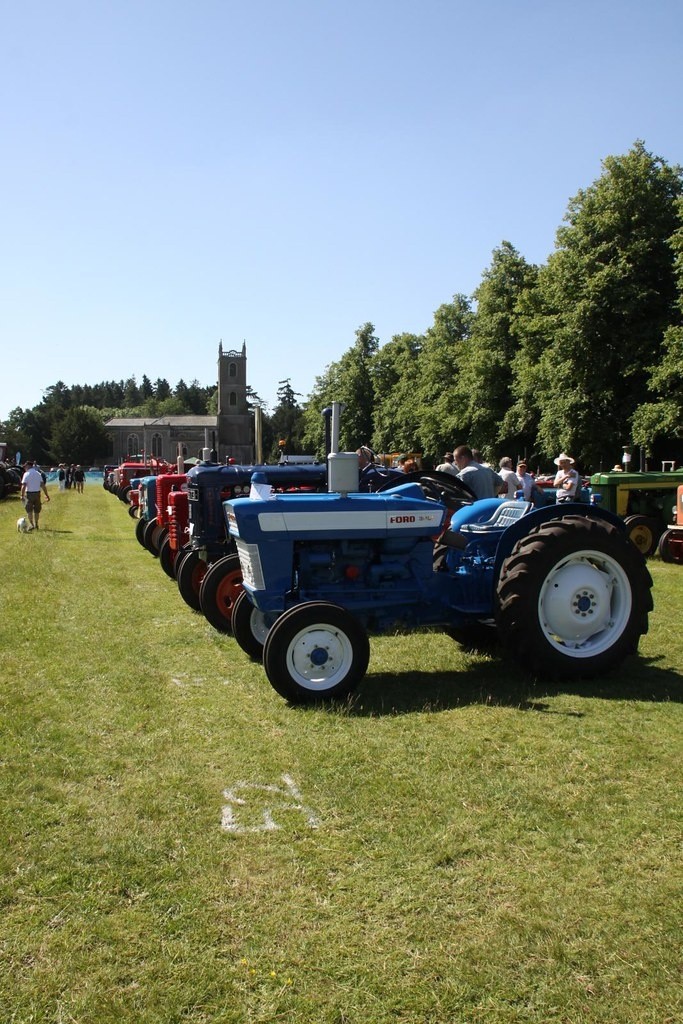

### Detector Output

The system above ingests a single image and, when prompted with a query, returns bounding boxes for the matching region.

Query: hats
[443,451,454,463]
[553,453,575,466]
[517,460,527,467]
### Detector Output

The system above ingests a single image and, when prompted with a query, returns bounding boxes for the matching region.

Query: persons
[554,452,579,504]
[20,461,50,530]
[3,456,15,468]
[33,461,86,494]
[356,445,543,504]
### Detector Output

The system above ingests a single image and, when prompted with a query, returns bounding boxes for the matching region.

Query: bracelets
[22,495,24,497]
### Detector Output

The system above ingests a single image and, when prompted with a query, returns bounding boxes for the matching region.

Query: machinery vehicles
[222,400,655,707]
[0,442,28,500]
[102,420,683,634]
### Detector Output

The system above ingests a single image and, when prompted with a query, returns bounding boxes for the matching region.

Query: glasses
[519,466,526,468]
[23,465,26,468]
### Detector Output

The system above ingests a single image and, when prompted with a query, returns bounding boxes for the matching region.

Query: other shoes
[34,525,38,528]
[27,525,34,531]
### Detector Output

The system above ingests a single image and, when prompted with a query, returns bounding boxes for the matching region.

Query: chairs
[459,500,534,551]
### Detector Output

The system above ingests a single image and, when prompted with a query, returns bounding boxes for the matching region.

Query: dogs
[16,517,29,533]
